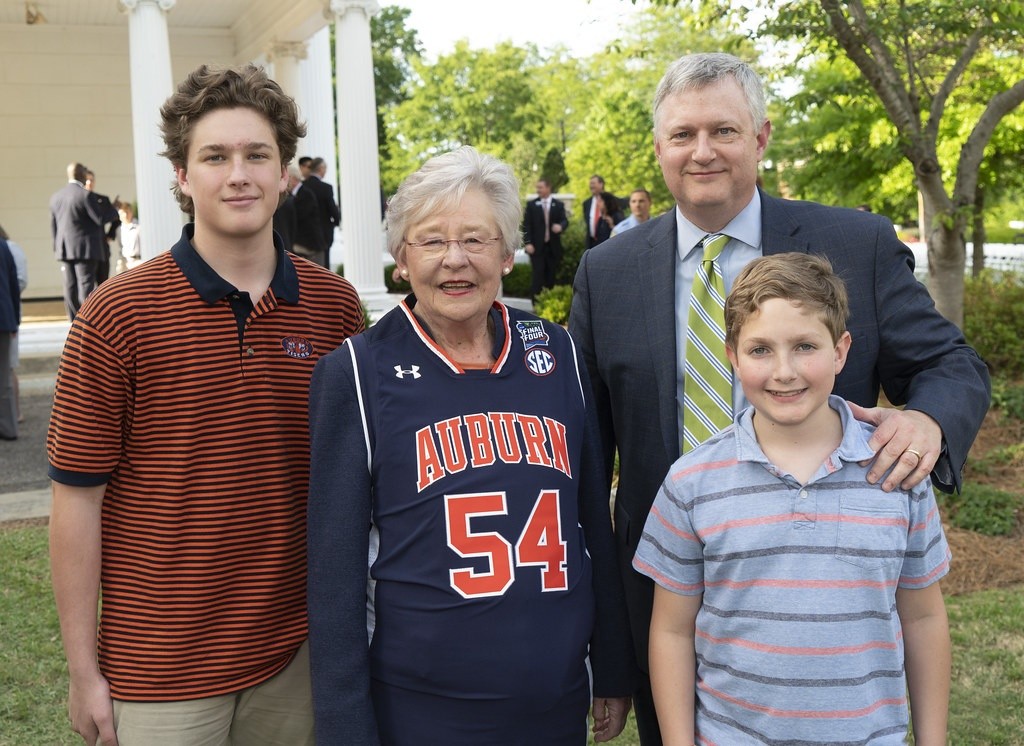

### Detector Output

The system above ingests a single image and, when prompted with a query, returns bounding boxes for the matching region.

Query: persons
[608,189,654,241]
[569,49,996,746]
[0,223,26,443]
[595,191,625,248]
[522,176,570,314]
[632,250,954,746]
[45,62,372,746]
[305,147,631,746]
[582,175,621,249]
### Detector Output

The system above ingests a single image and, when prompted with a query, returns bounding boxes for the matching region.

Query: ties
[594,197,600,236]
[683,233,732,455]
[544,200,549,243]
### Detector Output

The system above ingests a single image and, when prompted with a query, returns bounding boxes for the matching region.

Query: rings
[904,448,922,463]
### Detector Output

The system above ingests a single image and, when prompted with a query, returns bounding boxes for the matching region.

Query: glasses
[404,236,502,259]
